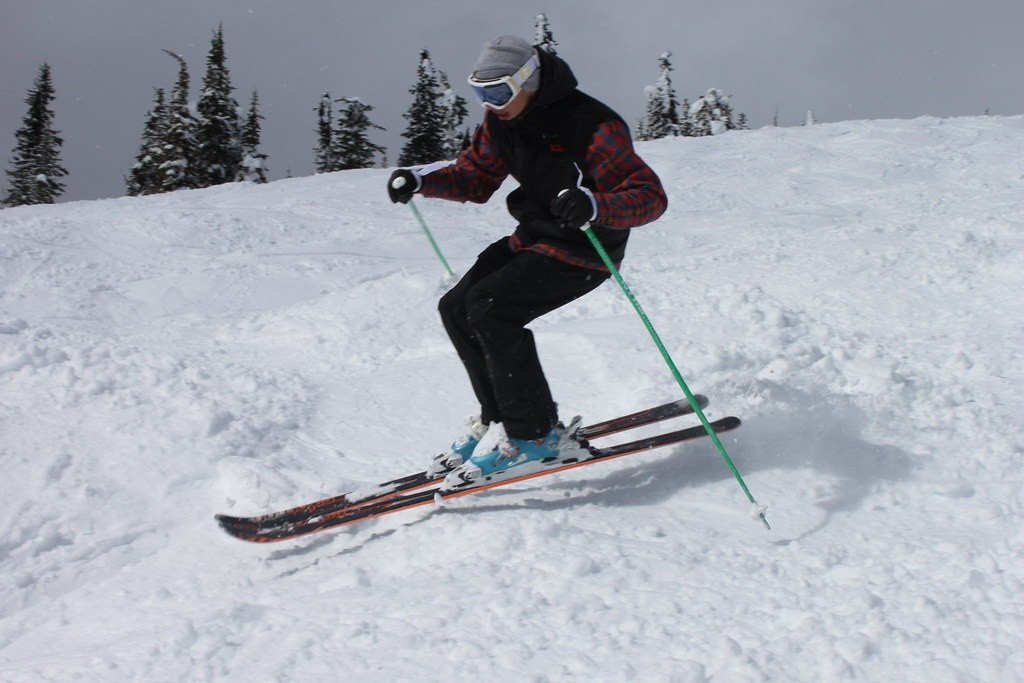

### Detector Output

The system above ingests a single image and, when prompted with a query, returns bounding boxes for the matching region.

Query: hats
[473,35,540,91]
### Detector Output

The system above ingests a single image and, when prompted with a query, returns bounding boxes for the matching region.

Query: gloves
[550,187,594,230]
[388,169,418,205]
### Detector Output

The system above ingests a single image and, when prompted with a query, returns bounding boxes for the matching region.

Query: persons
[386,36,668,491]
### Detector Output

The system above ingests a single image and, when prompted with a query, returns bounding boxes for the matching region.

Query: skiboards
[211,392,743,546]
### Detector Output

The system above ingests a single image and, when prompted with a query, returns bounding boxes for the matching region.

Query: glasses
[468,74,522,110]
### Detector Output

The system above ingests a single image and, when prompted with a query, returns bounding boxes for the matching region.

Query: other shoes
[440,415,491,468]
[444,414,560,479]
